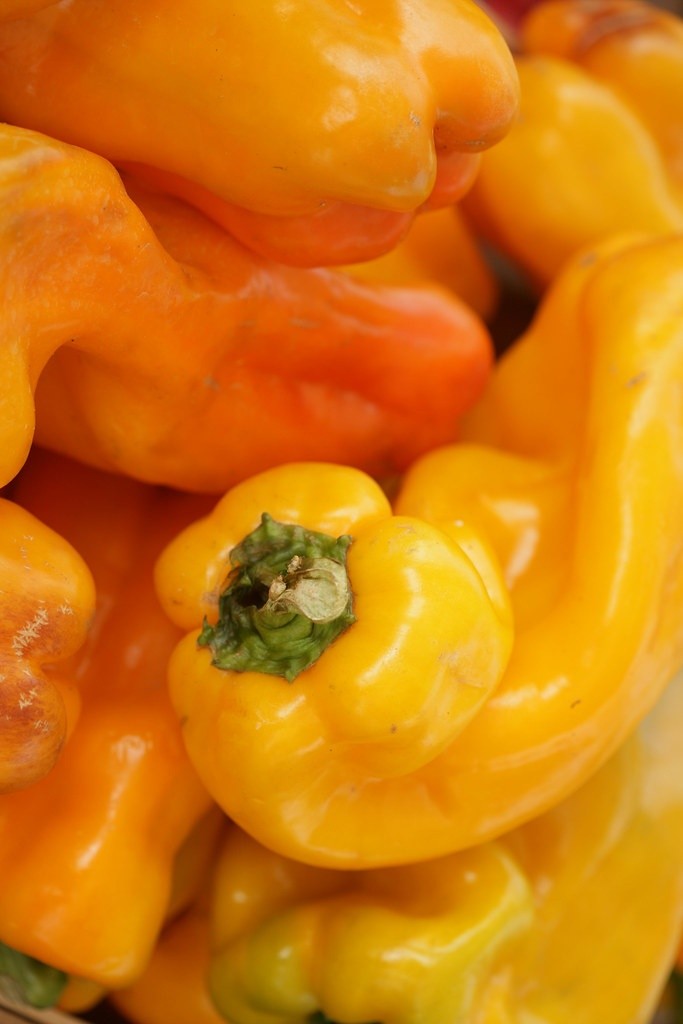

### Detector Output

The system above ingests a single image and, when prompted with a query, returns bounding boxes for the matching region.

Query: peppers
[0,0,683,1024]
